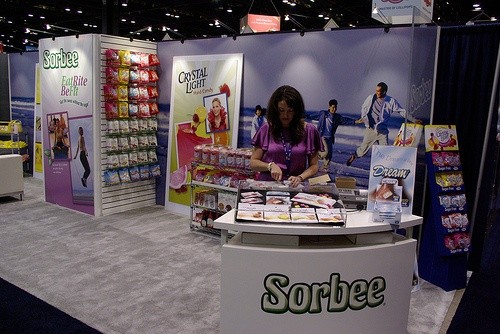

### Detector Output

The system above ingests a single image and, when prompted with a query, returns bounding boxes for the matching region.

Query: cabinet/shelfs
[189,161,237,239]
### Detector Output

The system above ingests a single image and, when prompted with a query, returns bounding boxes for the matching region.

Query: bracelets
[269,163,274,171]
[298,176,305,181]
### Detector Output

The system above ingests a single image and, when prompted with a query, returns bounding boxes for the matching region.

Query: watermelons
[170,165,187,189]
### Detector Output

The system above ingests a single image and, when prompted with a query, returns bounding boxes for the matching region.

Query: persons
[48,115,55,133]
[208,98,228,132]
[251,104,263,142]
[251,85,326,188]
[306,99,365,172]
[53,115,57,124]
[52,118,69,154]
[73,126,91,187]
[59,114,68,135]
[346,82,423,167]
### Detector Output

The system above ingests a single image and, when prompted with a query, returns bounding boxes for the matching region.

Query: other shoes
[346,154,355,166]
[81,178,87,188]
[324,168,330,173]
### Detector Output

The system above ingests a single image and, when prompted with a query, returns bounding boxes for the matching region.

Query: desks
[213,209,424,334]
